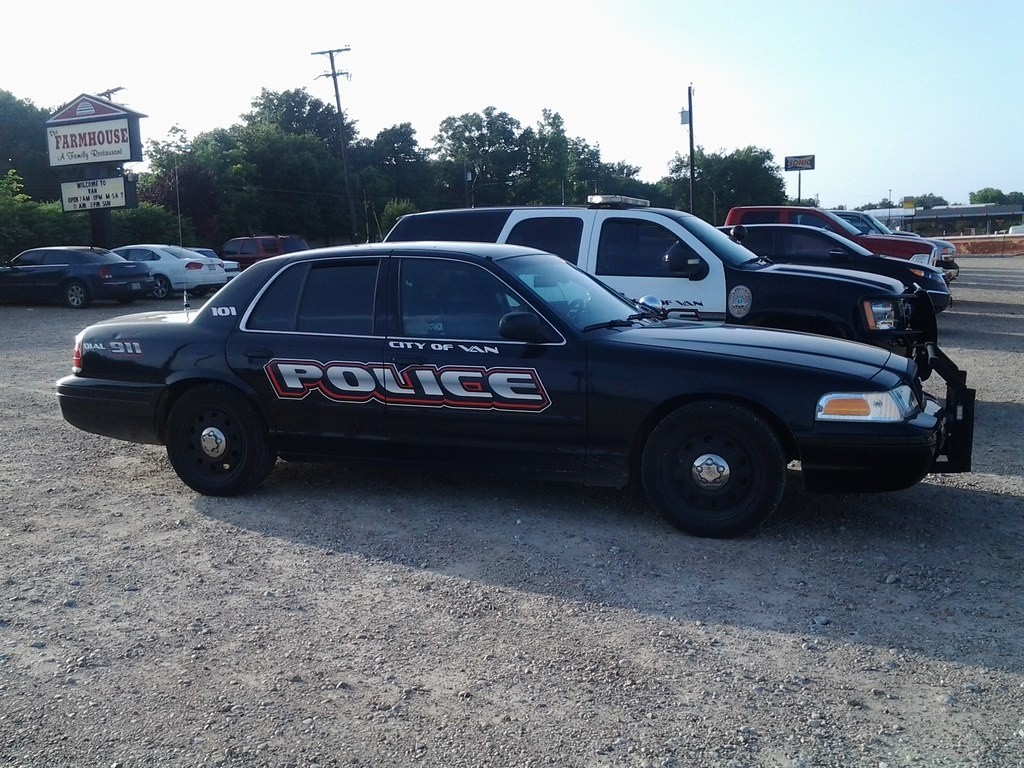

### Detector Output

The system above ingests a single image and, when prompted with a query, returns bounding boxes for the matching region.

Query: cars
[0,245,156,311]
[53,240,950,541]
[183,245,243,284]
[106,243,228,299]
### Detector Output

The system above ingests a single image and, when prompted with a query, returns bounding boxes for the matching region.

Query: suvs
[340,194,939,387]
[722,203,964,287]
[218,236,312,271]
[659,221,953,317]
